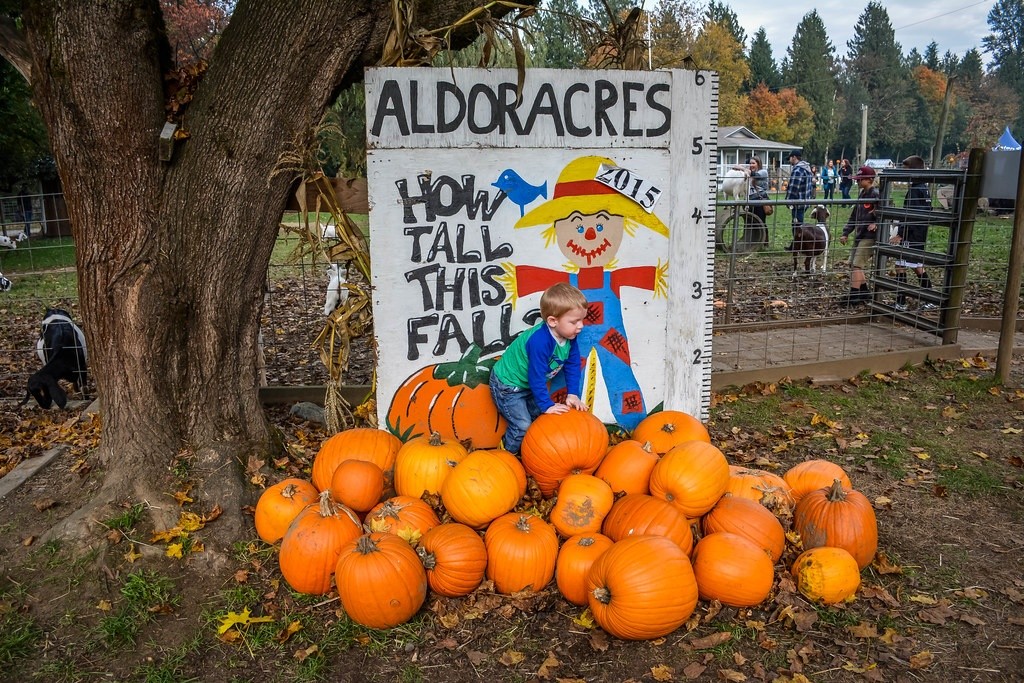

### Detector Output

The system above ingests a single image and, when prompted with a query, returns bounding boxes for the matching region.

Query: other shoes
[890,302,907,312]
[840,296,860,308]
[784,246,791,250]
[920,302,940,311]
[861,293,872,302]
[848,204,854,208]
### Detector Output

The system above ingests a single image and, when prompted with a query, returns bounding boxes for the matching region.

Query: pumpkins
[255,409,877,641]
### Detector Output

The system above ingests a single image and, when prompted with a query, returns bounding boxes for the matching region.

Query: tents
[991,125,1022,151]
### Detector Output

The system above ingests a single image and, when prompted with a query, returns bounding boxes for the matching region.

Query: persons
[884,156,940,313]
[839,166,879,307]
[785,149,814,252]
[820,158,838,209]
[17,183,38,238]
[838,159,854,209]
[809,165,818,199]
[488,282,589,463]
[733,156,769,247]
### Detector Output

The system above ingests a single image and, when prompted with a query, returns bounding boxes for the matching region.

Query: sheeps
[722,169,753,211]
[786,204,830,275]
[18,309,89,411]
[325,263,348,316]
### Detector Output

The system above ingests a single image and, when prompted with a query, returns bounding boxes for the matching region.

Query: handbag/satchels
[762,197,773,215]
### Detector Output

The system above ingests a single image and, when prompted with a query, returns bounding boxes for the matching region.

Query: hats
[785,149,801,160]
[853,167,876,180]
